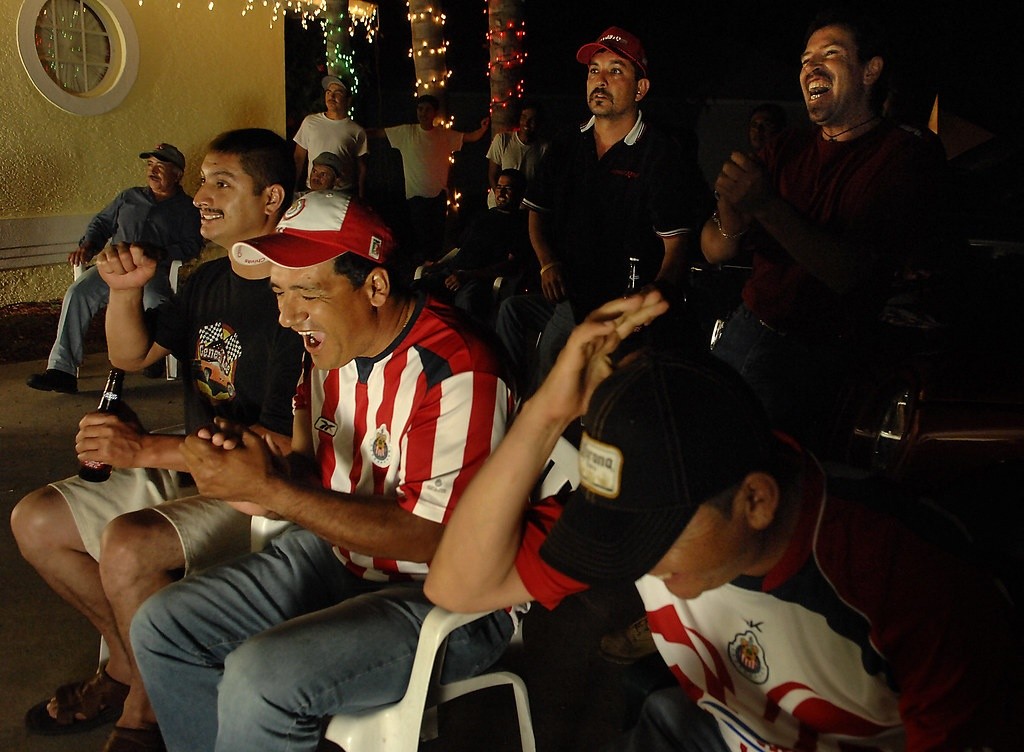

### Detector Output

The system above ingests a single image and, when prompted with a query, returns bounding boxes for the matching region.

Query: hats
[232,189,393,269]
[322,76,353,94]
[139,144,186,171]
[313,152,345,177]
[536,348,763,591]
[575,27,650,78]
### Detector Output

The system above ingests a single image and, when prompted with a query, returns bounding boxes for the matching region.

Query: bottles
[625,257,648,300]
[844,350,925,478]
[78,368,125,482]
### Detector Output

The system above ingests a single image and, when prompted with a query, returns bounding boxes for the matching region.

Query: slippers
[24,655,131,736]
[105,720,166,752]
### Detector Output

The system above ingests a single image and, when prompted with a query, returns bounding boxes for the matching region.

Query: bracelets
[540,260,559,275]
[713,208,750,239]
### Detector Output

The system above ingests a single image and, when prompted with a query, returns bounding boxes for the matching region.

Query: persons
[10,8,1013,752]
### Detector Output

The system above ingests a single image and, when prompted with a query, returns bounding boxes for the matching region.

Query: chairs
[73,259,183,381]
[324,433,581,752]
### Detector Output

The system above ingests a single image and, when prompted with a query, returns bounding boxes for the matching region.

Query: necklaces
[822,115,878,143]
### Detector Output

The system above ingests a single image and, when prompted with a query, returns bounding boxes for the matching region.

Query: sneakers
[145,355,167,378]
[27,369,79,393]
